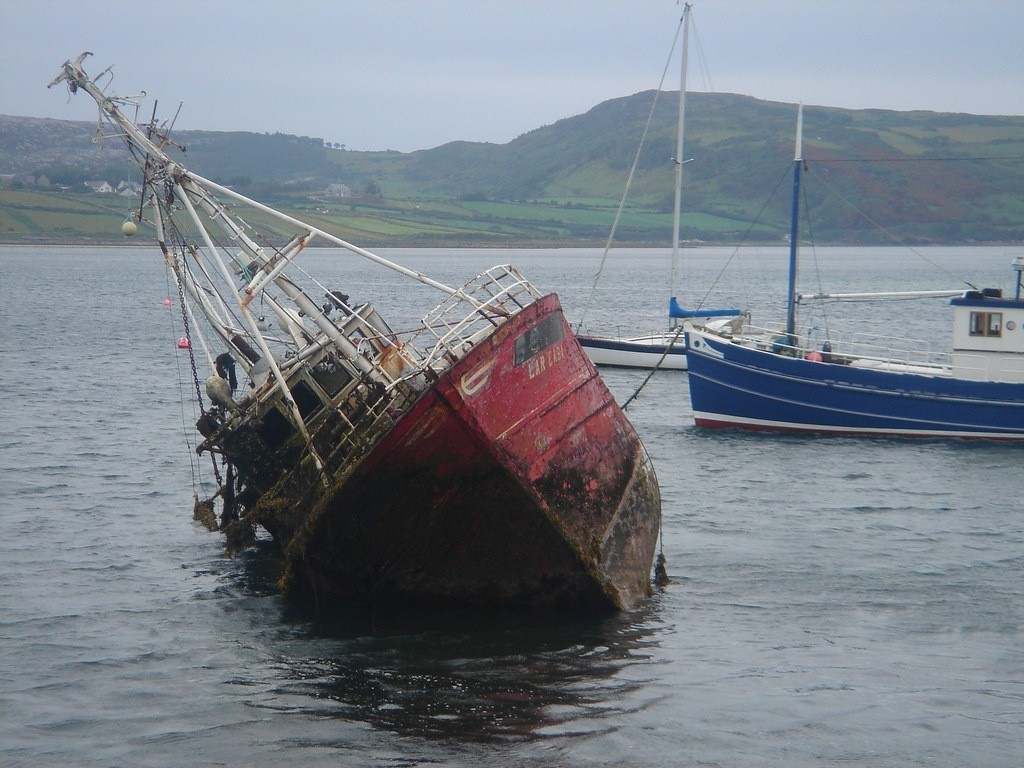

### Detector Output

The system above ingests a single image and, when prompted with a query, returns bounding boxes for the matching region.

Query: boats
[44,49,672,639]
[667,2,1023,445]
[573,318,734,370]
